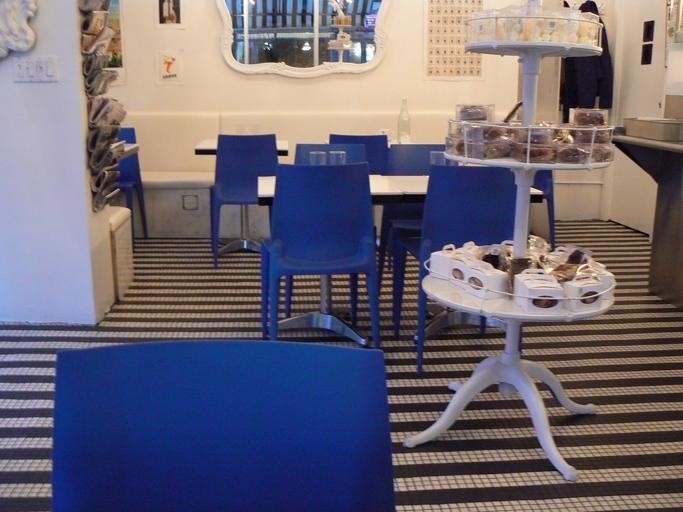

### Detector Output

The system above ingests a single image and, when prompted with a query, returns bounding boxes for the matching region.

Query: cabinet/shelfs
[396,1,624,484]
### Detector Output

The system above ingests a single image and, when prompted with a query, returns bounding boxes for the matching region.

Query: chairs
[206,132,281,270]
[258,162,381,350]
[392,163,518,378]
[328,134,389,175]
[529,170,556,252]
[378,143,461,294]
[114,125,149,252]
[284,142,364,329]
[48,338,395,512]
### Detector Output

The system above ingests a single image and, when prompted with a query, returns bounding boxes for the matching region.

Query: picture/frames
[156,48,186,86]
[155,0,184,29]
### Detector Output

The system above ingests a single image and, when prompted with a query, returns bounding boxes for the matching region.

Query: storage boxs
[622,117,683,142]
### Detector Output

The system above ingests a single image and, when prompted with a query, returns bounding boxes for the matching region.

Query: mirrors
[214,1,391,77]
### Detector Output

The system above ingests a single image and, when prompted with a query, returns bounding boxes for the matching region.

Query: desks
[192,141,290,157]
[257,176,545,348]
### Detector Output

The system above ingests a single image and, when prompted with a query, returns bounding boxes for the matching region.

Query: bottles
[396,96,411,145]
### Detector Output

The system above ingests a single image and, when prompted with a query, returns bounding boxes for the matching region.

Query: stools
[109,204,134,304]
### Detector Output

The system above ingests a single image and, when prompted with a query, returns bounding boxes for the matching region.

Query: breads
[448,104,613,164]
[425,247,616,308]
[328,16,351,49]
